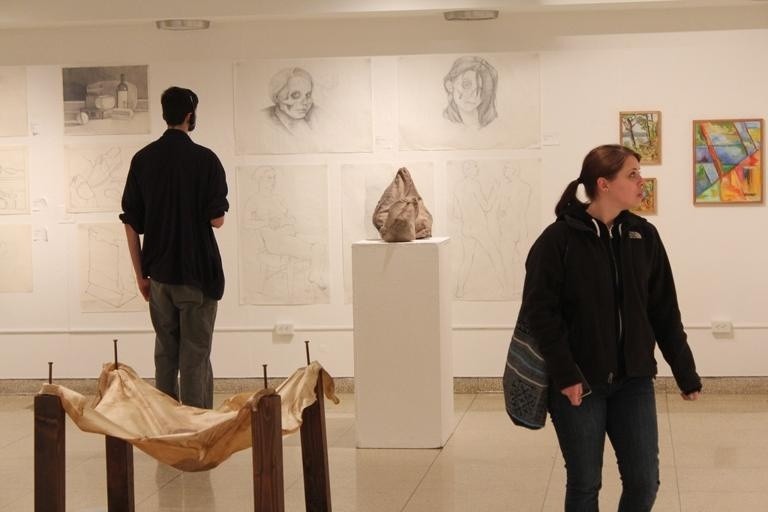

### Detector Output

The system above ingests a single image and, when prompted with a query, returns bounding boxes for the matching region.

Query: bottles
[115,73,128,110]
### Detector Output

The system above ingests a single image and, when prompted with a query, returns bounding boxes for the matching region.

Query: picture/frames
[629,178,658,216]
[619,111,663,166]
[693,118,763,204]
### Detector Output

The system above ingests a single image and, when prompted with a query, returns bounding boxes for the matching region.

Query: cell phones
[575,364,592,399]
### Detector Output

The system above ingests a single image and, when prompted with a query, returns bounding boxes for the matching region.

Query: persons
[260,67,326,135]
[120,87,229,409]
[525,145,702,511]
[442,56,498,129]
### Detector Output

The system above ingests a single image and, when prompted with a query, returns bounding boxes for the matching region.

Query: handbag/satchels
[503,308,549,430]
